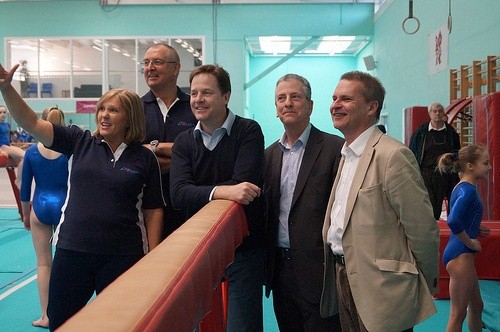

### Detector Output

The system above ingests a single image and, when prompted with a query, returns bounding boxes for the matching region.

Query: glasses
[143,58,177,67]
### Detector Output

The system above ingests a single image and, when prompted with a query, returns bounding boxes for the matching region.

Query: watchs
[151,140,159,151]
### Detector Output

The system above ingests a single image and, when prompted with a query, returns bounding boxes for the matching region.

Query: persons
[408,103,460,222]
[258,74,347,332]
[169,64,263,332]
[317,71,439,332]
[434,143,492,332]
[0,65,164,332]
[131,45,198,243]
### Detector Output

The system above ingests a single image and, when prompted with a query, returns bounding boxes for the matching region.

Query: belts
[277,248,291,260]
[331,255,345,265]
